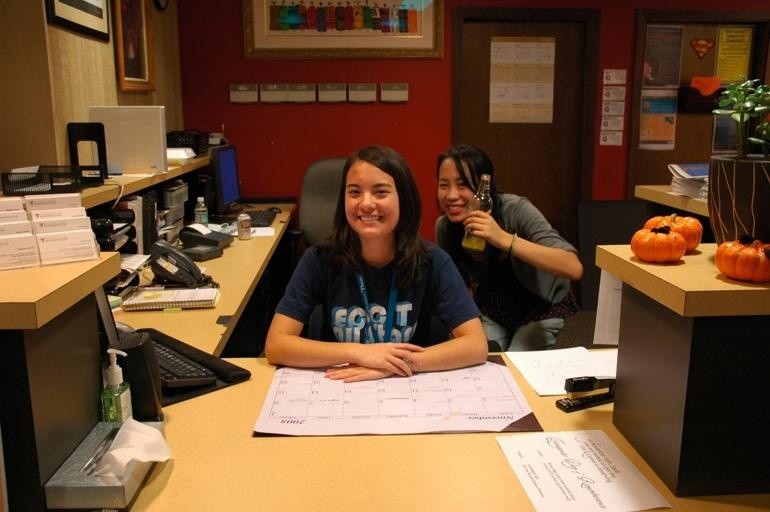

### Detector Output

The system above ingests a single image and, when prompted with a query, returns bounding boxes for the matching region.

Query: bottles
[195,197,208,228]
[461,174,493,253]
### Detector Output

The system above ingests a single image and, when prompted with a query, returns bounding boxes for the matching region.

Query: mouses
[268,206,281,213]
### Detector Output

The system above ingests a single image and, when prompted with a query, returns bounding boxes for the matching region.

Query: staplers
[556,375,618,412]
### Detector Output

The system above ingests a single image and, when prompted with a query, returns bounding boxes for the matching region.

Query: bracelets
[509,232,517,254]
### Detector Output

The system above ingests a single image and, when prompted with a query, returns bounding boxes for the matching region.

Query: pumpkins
[715,238,770,282]
[631,229,685,262]
[643,213,704,252]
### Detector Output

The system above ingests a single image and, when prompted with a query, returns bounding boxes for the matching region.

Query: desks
[634,184,713,243]
[1,242,770,512]
[1,149,297,356]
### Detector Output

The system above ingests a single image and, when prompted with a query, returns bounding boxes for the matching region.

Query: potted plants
[707,77,770,245]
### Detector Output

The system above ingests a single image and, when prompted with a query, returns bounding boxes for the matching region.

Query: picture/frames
[47,0,111,43]
[242,1,445,62]
[114,0,157,93]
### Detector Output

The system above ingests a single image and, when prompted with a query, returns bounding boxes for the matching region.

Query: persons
[435,146,584,353]
[265,146,489,383]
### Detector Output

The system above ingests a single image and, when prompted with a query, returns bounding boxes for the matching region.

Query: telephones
[152,239,220,289]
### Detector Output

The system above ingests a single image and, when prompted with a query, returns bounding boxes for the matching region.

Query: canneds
[238,214,251,240]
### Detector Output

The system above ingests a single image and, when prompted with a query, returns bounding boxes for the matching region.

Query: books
[123,288,220,312]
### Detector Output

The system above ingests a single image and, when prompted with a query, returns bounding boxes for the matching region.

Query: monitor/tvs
[212,145,241,211]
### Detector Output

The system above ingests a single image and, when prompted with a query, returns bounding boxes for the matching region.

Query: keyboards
[152,340,218,390]
[245,209,276,226]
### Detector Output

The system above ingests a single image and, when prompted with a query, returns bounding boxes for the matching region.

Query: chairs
[290,157,348,263]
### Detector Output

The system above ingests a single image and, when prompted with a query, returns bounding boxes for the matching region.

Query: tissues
[44,420,176,509]
